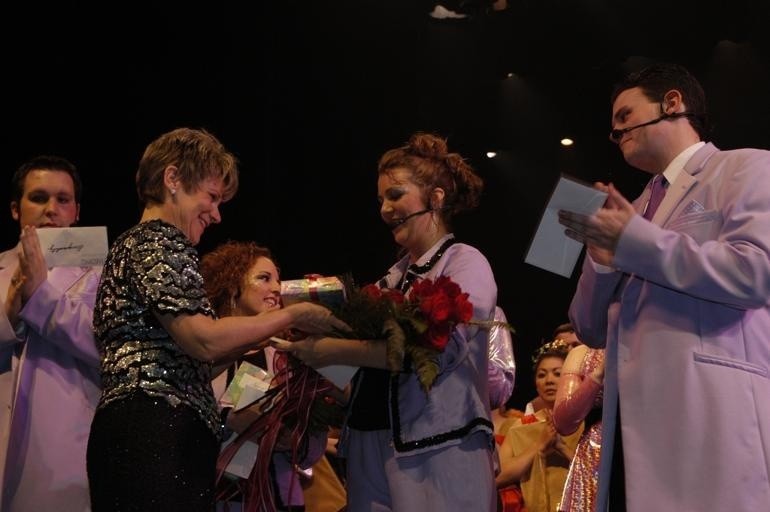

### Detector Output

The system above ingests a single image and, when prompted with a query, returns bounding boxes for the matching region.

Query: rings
[10,276,18,284]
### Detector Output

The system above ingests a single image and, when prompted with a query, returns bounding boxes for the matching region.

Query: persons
[557,63,770,512]
[86,126,353,512]
[195,241,327,512]
[301,306,607,508]
[271,132,498,512]
[0,155,114,512]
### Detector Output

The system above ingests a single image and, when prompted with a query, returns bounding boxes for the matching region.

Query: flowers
[286,274,516,399]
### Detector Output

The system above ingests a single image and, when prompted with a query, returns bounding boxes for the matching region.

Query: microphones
[612,111,696,139]
[385,193,433,232]
